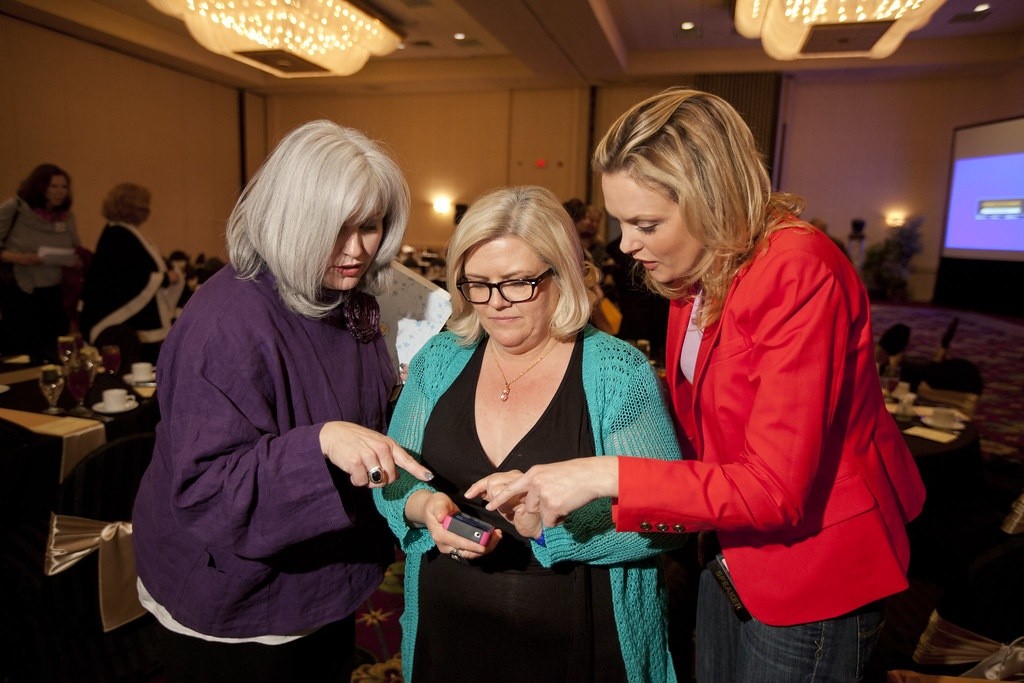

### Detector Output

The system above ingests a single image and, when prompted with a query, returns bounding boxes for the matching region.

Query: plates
[92,400,139,413]
[121,374,156,384]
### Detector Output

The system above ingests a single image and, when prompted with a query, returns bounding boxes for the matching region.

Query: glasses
[455,265,552,304]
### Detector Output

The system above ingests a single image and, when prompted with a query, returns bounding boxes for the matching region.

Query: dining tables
[885,390,976,470]
[0,354,156,509]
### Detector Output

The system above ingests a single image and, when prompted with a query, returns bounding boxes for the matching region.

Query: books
[361,260,453,388]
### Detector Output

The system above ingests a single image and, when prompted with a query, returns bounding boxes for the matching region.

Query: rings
[449,549,463,561]
[368,466,383,483]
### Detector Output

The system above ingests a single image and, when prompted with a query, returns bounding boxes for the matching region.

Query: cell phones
[442,510,497,547]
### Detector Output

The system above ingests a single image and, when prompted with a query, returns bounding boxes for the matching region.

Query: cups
[102,389,136,409]
[59,337,74,365]
[132,363,156,381]
[102,346,120,375]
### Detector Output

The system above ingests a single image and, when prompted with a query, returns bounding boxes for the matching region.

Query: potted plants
[860,213,925,306]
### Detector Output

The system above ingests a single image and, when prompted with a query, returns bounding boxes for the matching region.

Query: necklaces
[487,337,562,401]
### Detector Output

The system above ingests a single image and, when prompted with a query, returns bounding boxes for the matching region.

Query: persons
[561,197,670,369]
[371,184,682,683]
[0,163,229,373]
[132,120,437,683]
[486,85,928,683]
[397,245,446,287]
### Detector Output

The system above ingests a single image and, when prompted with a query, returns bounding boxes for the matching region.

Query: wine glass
[67,363,96,416]
[40,366,64,414]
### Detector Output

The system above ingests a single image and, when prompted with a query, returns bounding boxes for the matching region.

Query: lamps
[143,0,405,79]
[733,0,948,61]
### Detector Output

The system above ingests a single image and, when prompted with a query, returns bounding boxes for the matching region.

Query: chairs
[0,429,159,682]
